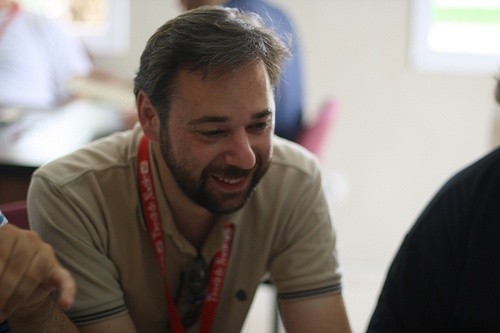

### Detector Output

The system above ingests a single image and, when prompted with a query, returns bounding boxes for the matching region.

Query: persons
[367,72,500,333]
[182,0,301,141]
[0,210,76,333]
[0,0,95,146]
[27,5,353,333]
